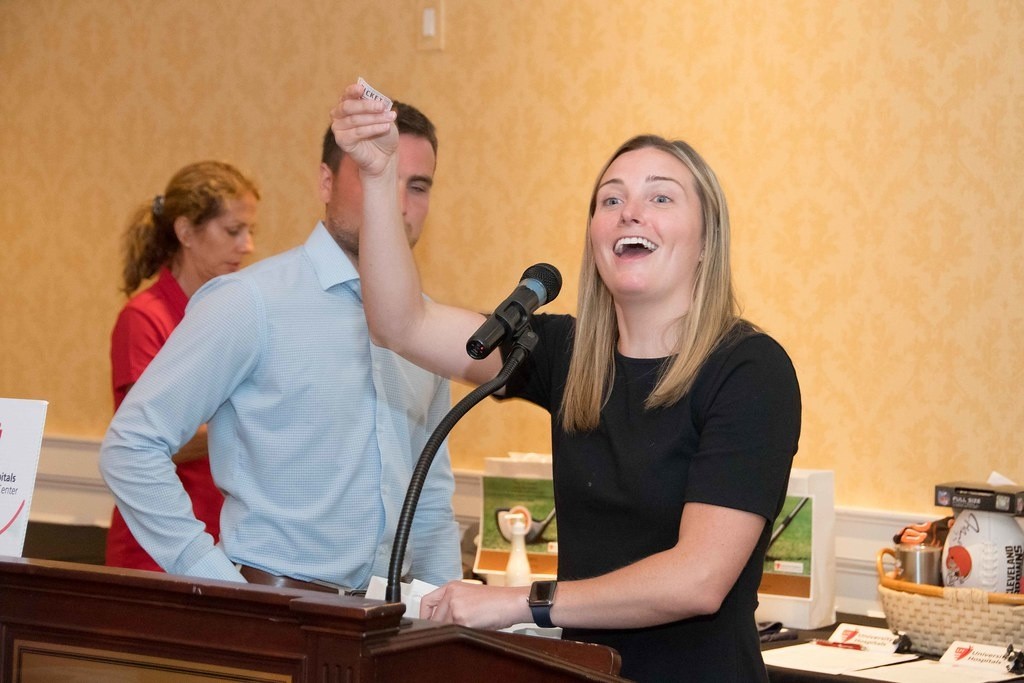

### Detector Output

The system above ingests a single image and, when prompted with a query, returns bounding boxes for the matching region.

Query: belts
[236,564,366,602]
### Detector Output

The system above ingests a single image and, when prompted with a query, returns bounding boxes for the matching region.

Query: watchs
[526,581,558,628]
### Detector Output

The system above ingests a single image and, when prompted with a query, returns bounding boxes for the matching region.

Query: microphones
[466,262,563,362]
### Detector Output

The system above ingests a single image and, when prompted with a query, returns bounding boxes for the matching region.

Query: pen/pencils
[812,638,867,651]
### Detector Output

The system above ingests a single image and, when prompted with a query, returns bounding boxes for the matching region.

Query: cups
[897,545,939,584]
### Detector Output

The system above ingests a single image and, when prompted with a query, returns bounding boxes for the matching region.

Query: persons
[105,160,262,571]
[327,85,801,683]
[104,100,460,597]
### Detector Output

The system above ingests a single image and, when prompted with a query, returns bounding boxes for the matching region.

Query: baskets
[876,547,1024,655]
